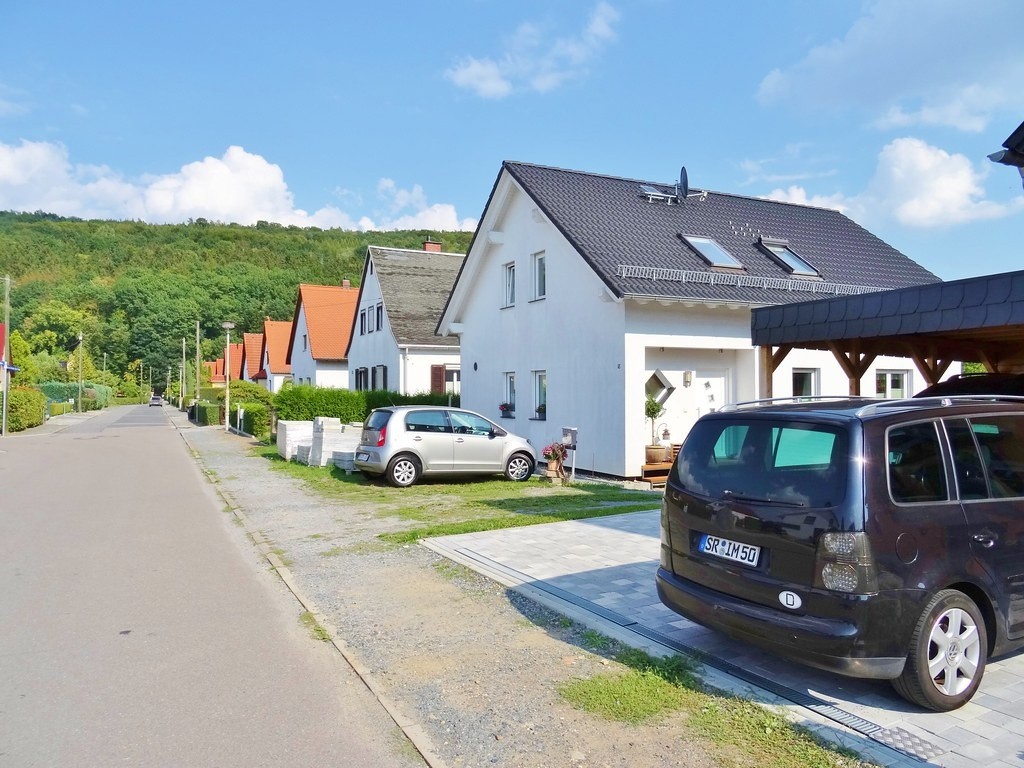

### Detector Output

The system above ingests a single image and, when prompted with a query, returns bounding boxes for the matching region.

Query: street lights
[221,320,235,433]
[178,364,184,398]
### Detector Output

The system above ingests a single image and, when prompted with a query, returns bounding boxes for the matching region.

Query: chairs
[965,445,1014,497]
[828,462,912,501]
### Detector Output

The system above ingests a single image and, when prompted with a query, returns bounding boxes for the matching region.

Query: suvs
[655,372,1023,713]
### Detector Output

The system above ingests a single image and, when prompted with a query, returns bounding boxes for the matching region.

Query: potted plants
[645,393,665,464]
[536,404,546,419]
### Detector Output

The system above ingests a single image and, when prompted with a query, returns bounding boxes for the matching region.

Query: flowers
[543,442,568,461]
[498,402,511,411]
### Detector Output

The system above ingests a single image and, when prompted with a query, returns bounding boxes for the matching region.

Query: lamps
[684,370,691,382]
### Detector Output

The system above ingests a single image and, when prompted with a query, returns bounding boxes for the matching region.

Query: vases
[501,410,510,417]
[548,459,559,471]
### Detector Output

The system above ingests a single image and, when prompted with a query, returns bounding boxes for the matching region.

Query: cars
[353,405,537,487]
[149,396,163,407]
